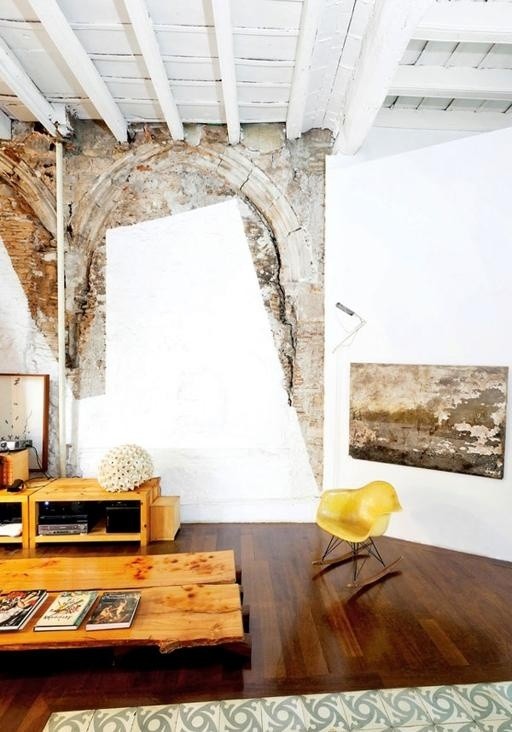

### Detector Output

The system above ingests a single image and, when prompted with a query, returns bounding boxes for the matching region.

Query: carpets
[43,680,512,732]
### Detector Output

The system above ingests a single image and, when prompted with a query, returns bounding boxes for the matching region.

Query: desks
[1,548,253,658]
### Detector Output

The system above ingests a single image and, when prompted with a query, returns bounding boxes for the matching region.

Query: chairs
[311,480,403,589]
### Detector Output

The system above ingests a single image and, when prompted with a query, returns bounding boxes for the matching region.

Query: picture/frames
[0,372,50,471]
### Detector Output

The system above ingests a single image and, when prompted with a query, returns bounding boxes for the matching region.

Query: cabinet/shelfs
[29,477,162,554]
[0,476,58,550]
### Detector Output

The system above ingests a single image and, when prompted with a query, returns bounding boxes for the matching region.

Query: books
[85,591,141,631]
[32,591,99,631]
[0,588,50,631]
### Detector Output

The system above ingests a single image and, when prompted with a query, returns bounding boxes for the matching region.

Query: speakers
[106,501,140,533]
